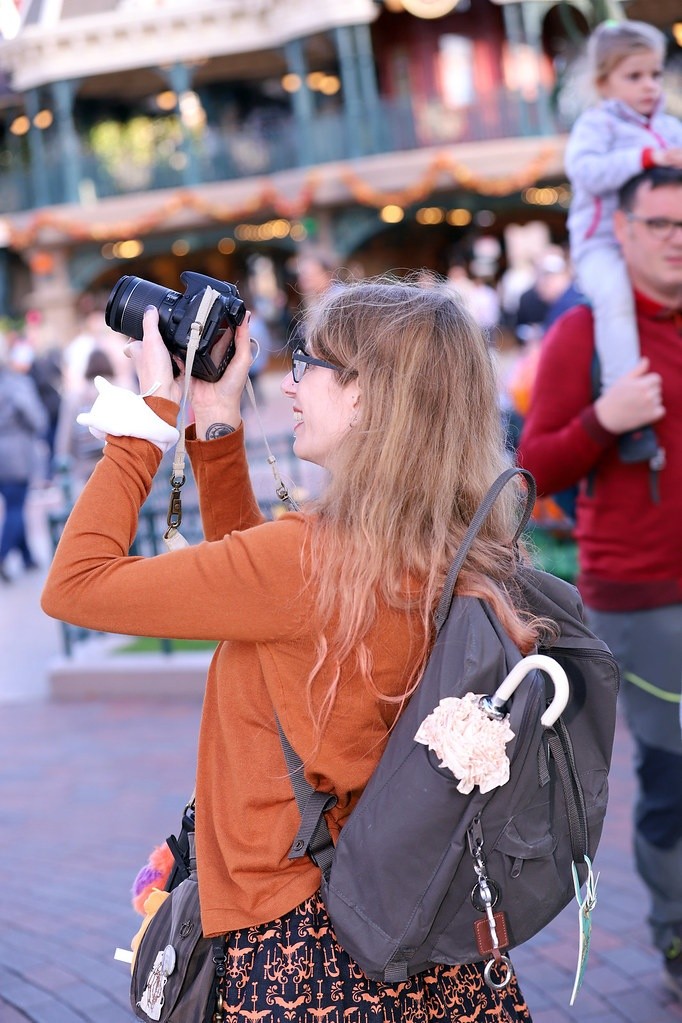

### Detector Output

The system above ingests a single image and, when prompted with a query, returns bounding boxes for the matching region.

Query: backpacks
[286,465,626,1009]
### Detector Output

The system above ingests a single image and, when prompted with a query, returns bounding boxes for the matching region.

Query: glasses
[629,214,681,241]
[290,345,358,384]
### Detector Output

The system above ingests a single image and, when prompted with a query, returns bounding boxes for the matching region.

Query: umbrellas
[412,655,569,794]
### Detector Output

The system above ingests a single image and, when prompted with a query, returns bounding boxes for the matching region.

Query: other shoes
[617,423,657,463]
[661,963,682,1000]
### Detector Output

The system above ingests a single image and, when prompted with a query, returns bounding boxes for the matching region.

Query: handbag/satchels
[130,823,221,1022]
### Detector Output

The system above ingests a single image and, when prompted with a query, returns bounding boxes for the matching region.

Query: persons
[0,22,682,1022]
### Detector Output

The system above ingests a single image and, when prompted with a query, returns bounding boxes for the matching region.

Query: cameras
[104,273,246,383]
